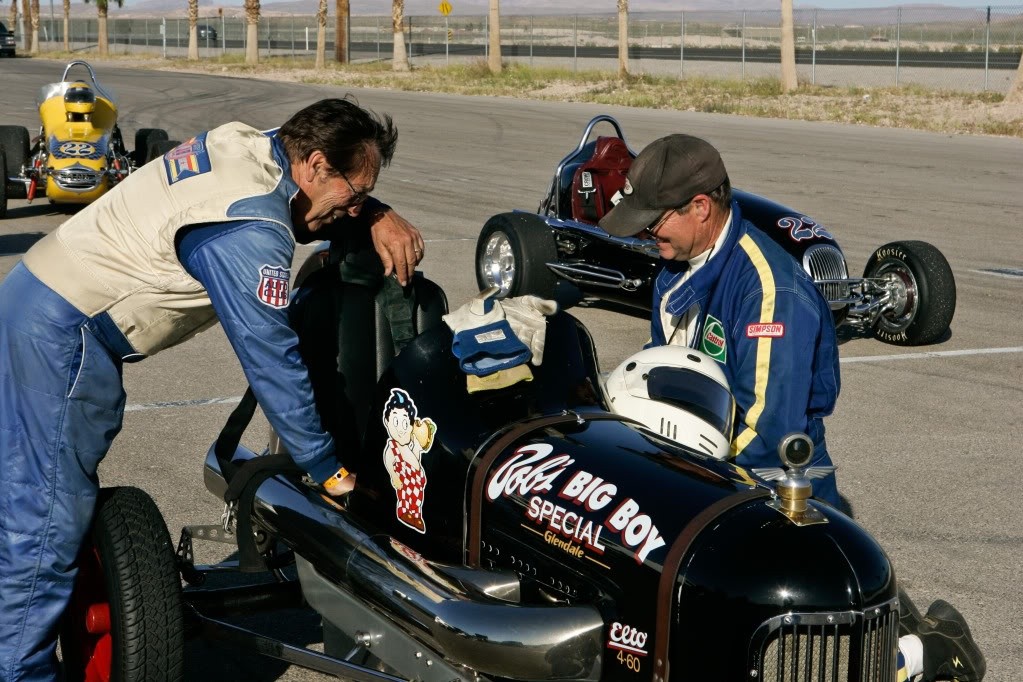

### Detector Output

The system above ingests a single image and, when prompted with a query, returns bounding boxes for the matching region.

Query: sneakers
[896,586,922,638]
[911,598,986,682]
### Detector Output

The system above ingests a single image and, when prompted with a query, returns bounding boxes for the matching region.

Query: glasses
[331,163,369,206]
[646,207,677,238]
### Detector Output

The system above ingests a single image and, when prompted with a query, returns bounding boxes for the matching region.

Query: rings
[415,249,422,252]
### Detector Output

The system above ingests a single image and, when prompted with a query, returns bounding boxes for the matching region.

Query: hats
[599,133,726,238]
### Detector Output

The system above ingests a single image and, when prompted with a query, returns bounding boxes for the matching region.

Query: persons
[0,98,425,682]
[597,133,841,508]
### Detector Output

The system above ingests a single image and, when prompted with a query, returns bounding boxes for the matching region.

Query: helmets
[605,344,737,462]
[64,82,96,114]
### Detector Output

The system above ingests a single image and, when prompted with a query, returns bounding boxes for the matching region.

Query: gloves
[441,286,558,393]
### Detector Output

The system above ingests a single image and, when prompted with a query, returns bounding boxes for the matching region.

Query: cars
[0,58,182,219]
[197,25,217,41]
[53,219,986,682]
[0,22,16,57]
[475,114,958,350]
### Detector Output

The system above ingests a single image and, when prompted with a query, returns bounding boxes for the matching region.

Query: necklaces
[667,245,715,345]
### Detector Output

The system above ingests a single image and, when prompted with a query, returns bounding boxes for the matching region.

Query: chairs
[570,136,633,225]
[281,220,450,462]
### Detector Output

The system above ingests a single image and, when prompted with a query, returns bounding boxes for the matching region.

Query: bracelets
[323,466,349,488]
